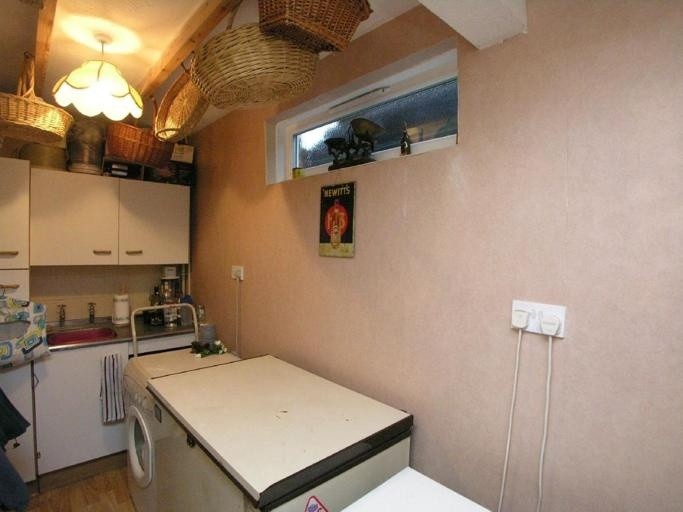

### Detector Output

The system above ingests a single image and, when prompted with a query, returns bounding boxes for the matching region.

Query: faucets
[87,302,96,324]
[56,303,67,328]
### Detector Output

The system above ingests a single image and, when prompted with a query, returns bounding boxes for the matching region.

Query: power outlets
[231,265,244,281]
[512,300,566,339]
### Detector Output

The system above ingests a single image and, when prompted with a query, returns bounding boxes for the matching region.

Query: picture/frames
[320,181,355,258]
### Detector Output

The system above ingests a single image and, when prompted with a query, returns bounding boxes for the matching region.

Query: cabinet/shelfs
[30,169,190,265]
[0,156,30,303]
[33,342,133,476]
[1,361,37,484]
[130,333,199,360]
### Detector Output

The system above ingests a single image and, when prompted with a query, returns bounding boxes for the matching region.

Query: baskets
[0,55,74,144]
[189,6,319,110]
[107,92,171,169]
[154,68,207,143]
[258,1,373,53]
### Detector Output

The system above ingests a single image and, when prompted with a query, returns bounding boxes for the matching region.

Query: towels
[99,351,126,424]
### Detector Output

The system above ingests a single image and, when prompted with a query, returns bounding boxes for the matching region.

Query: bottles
[151,284,163,326]
[330,199,340,249]
[401,121,411,156]
[180,295,192,326]
[162,281,170,300]
[164,302,177,327]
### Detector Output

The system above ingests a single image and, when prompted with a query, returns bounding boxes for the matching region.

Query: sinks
[46,321,121,348]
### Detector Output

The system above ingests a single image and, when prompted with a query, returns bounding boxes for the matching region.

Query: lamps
[51,41,143,121]
